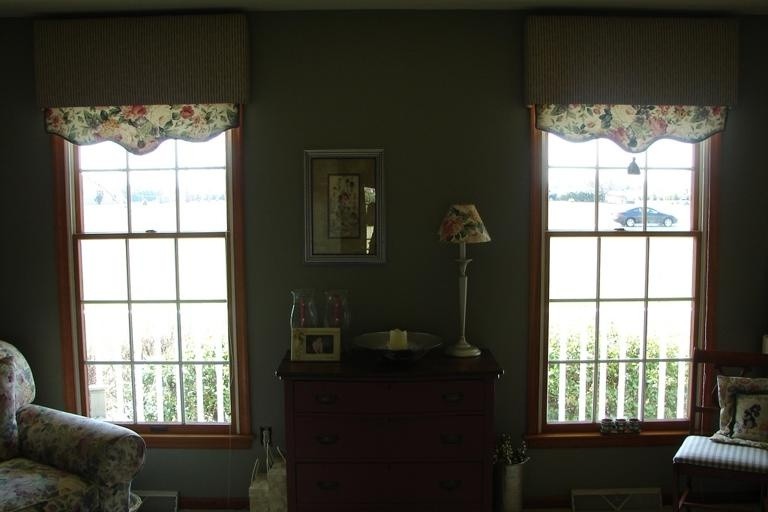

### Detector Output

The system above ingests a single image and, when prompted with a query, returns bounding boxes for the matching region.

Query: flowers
[439,205,473,243]
[491,428,532,465]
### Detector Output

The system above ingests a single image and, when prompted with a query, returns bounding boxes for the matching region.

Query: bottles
[627,418,640,431]
[601,418,613,432]
[615,418,627,432]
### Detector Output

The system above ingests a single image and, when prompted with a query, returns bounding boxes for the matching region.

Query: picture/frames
[302,148,386,263]
[290,327,341,363]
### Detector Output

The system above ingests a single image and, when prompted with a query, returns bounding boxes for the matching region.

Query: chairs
[0,340,148,512]
[671,347,767,512]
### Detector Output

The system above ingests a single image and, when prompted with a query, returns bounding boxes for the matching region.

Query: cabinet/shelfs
[276,342,503,512]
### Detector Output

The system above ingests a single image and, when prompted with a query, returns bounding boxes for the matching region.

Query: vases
[503,463,522,512]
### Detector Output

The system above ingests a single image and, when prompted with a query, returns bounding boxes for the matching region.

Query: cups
[320,288,352,354]
[287,288,317,330]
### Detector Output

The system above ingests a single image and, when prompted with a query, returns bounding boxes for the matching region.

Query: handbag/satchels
[249,443,287,512]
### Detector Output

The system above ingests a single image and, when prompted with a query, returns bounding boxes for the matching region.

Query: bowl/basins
[351,330,444,360]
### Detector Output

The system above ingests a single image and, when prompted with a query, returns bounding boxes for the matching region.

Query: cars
[613,206,678,228]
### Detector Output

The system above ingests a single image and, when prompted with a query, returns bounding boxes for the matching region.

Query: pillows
[710,374,767,451]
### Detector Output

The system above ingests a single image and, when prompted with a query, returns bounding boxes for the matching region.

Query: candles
[334,296,340,326]
[388,326,408,348]
[300,295,305,327]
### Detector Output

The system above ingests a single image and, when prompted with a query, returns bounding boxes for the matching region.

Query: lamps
[435,200,491,357]
